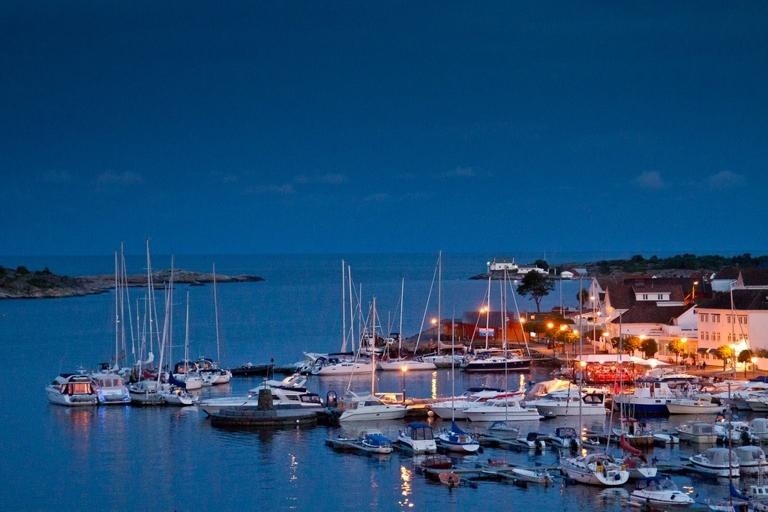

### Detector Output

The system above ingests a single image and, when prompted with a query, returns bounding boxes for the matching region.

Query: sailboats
[293,248,612,422]
[561,273,630,486]
[109,238,232,407]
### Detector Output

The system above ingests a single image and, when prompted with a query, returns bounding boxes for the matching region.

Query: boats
[681,447,741,479]
[438,426,480,453]
[467,413,768,454]
[612,371,768,415]
[734,445,767,477]
[395,423,438,455]
[359,438,394,455]
[45,360,98,407]
[627,474,696,508]
[512,468,554,483]
[89,368,132,404]
[625,466,660,482]
[191,369,325,427]
